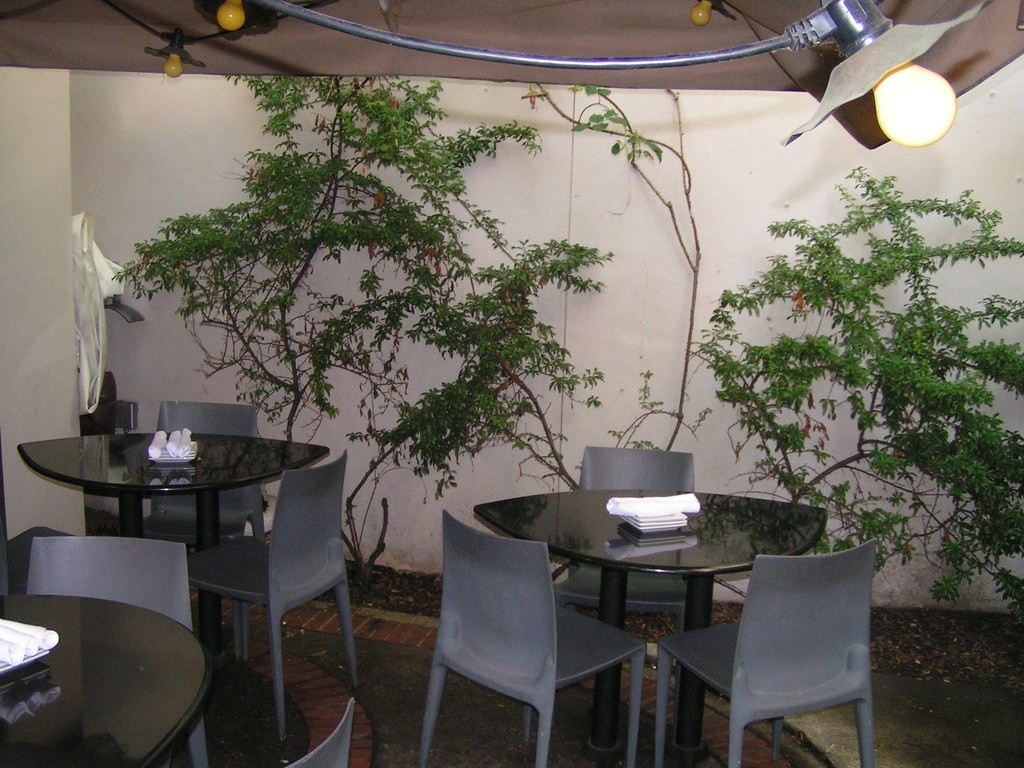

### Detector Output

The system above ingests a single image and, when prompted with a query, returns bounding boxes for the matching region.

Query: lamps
[778,0,995,149]
[144,28,205,78]
[689,0,737,27]
[193,0,278,34]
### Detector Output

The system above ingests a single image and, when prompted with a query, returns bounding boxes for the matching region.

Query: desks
[0,594,213,768]
[474,491,828,768]
[17,432,331,768]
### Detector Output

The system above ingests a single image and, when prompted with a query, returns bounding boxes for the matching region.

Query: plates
[0,648,49,675]
[620,513,689,531]
[148,440,199,463]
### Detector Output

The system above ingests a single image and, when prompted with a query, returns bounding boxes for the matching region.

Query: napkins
[0,618,59,665]
[605,492,701,514]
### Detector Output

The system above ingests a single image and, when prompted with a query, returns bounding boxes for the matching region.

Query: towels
[0,625,39,657]
[0,618,60,650]
[166,430,182,458]
[178,428,192,458]
[148,430,167,459]
[0,640,25,666]
[606,492,702,517]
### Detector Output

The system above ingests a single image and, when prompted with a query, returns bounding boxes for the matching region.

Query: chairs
[26,536,209,767]
[653,538,876,768]
[418,508,648,768]
[285,696,356,768]
[0,517,72,595]
[553,446,695,722]
[188,448,358,741]
[143,402,264,660]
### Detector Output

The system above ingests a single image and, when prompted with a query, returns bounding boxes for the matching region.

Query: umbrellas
[0,0,1024,148]
